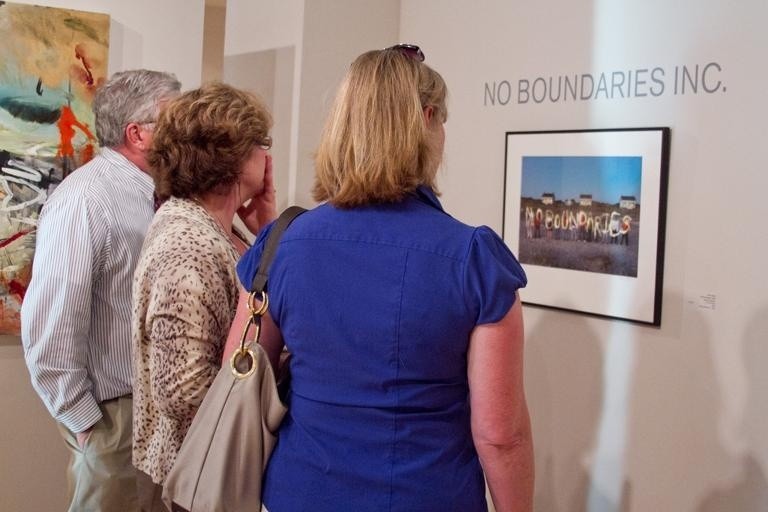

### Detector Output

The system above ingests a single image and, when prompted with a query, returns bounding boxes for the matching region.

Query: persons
[525,205,633,246]
[220,41,536,512]
[130,79,280,512]
[19,67,183,512]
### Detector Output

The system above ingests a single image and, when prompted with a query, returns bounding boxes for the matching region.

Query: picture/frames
[499,124,671,329]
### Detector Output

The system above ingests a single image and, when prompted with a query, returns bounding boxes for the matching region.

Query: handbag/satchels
[161,339,288,511]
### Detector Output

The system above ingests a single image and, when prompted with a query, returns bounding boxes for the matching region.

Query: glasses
[386,43,425,62]
[253,136,272,150]
